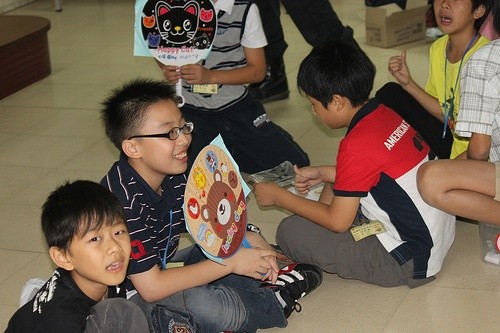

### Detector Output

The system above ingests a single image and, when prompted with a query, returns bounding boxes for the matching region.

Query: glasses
[127,120,194,141]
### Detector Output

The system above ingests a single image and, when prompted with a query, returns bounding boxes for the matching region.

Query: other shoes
[246,67,289,104]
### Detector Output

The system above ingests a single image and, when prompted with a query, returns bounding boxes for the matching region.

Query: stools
[0,15,51,99]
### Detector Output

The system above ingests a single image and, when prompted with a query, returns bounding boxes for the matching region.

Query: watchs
[247,224,261,233]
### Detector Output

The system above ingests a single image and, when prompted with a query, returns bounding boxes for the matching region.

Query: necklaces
[439,34,477,138]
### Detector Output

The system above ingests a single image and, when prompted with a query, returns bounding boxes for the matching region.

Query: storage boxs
[354,2,433,47]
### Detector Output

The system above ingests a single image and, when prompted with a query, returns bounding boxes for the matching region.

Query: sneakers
[259,261,324,318]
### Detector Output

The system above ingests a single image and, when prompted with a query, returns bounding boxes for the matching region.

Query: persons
[154,0,311,174]
[416,0,500,227]
[253,38,456,287]
[249,0,346,103]
[99,79,323,333]
[4,180,196,333]
[388,0,494,159]
